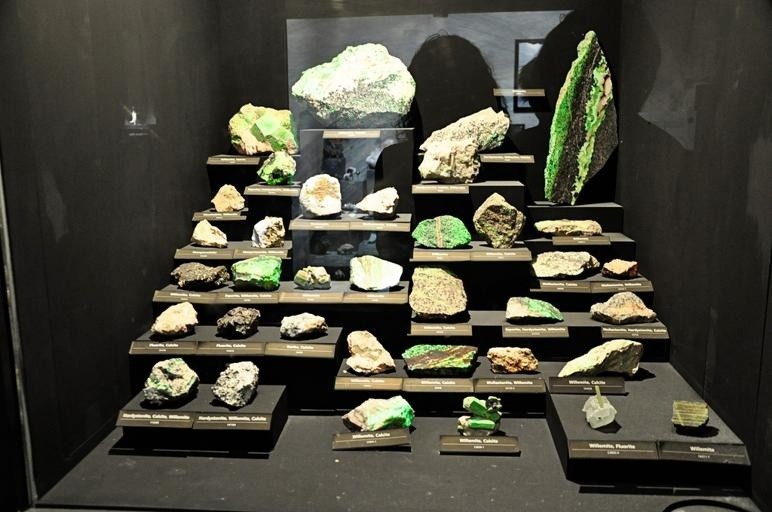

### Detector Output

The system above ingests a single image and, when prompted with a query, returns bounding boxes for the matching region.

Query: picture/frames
[513,38,551,114]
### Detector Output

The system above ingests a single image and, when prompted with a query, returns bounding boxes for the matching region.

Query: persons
[512,8,613,199]
[372,33,522,258]
